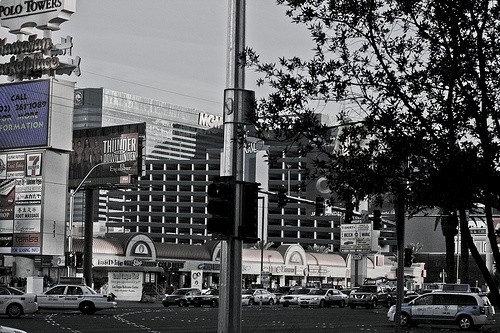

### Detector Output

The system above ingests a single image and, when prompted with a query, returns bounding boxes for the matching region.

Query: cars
[279,288,319,307]
[0,286,38,318]
[241,289,277,306]
[190,289,220,307]
[36,284,118,314]
[298,288,350,308]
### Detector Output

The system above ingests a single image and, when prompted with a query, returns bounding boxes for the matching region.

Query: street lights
[67,162,108,277]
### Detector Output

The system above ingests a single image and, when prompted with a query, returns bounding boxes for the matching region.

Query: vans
[386,292,496,330]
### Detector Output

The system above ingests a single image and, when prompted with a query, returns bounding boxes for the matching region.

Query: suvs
[161,288,201,307]
[349,285,408,309]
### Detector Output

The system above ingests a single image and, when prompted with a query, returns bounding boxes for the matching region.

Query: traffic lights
[315,197,324,216]
[76,251,83,268]
[344,203,354,223]
[404,248,413,267]
[373,209,382,230]
[278,188,289,209]
[207,175,236,241]
